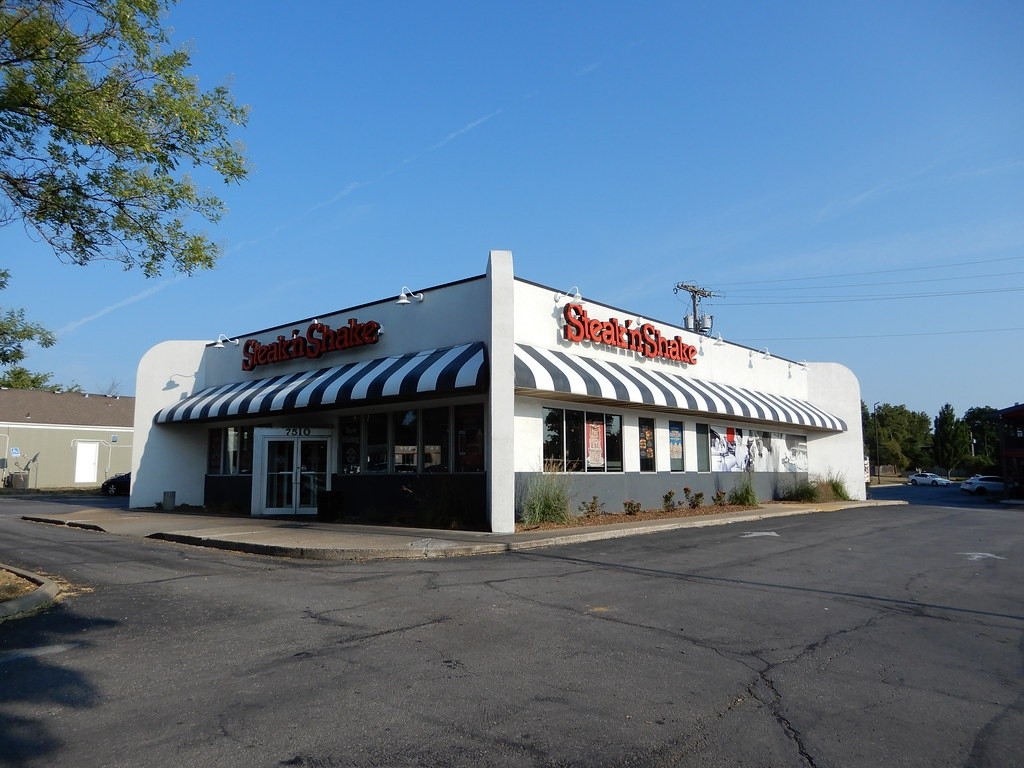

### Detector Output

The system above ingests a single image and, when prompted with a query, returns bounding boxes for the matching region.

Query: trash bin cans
[163,490,176,510]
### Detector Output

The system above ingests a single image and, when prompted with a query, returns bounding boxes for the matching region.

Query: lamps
[789,359,809,371]
[396,286,424,306]
[212,334,239,349]
[749,347,772,360]
[699,331,726,347]
[554,286,586,306]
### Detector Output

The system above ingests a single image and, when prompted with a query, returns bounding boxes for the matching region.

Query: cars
[909,472,950,487]
[971,474,981,480]
[102,472,131,495]
[960,476,1019,493]
